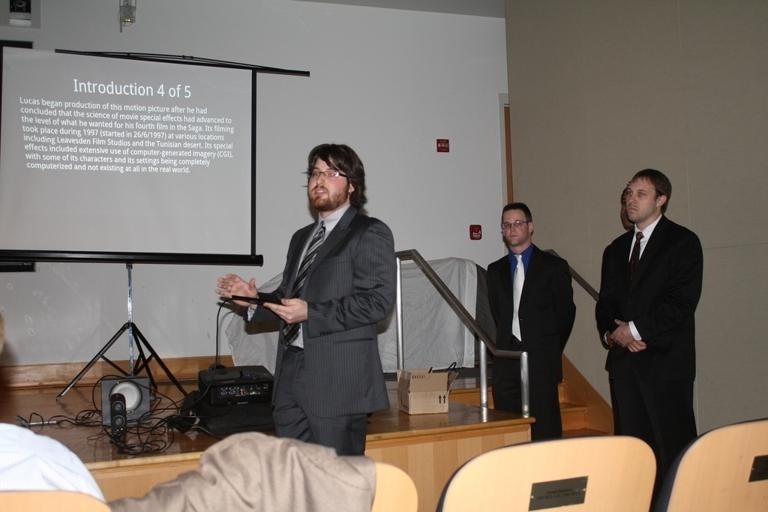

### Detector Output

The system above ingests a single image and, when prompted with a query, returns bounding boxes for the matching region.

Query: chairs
[652,416,768,511]
[436,435,658,512]
[0,490,112,512]
[370,462,419,512]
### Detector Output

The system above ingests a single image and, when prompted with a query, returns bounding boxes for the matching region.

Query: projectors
[198,364,275,407]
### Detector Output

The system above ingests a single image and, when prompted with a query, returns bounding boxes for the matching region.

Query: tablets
[230,295,283,305]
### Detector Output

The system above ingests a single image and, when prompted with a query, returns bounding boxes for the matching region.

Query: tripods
[57,268,189,400]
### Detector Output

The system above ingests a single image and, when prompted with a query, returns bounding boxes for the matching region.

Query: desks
[364,403,536,512]
[0,354,236,503]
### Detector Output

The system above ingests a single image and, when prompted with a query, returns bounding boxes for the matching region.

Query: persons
[487,203,575,442]
[596,168,703,512]
[620,190,634,230]
[214,145,397,457]
[0,313,107,502]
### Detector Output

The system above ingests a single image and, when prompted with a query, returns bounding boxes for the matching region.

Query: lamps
[119,0,137,33]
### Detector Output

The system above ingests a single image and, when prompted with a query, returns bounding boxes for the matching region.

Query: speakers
[110,394,127,438]
[100,375,152,426]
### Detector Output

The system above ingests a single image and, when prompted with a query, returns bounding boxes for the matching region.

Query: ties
[511,255,525,343]
[628,232,643,282]
[281,220,325,349]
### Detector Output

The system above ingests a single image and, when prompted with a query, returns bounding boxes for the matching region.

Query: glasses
[500,220,528,230]
[310,170,350,181]
